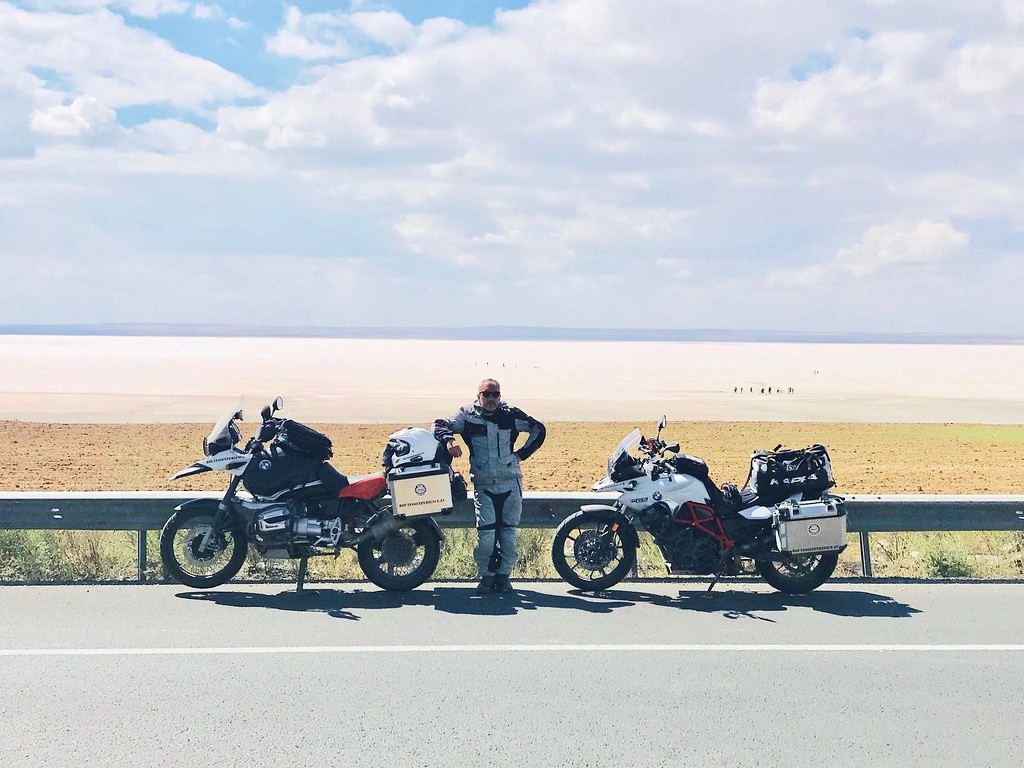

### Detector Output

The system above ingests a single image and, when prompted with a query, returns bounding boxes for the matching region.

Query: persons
[431,379,546,595]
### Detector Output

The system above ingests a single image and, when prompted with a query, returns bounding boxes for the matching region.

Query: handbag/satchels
[750,444,836,497]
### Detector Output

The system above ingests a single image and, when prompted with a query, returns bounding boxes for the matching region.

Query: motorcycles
[549,413,857,603]
[158,392,468,599]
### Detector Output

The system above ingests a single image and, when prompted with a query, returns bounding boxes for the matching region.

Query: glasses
[479,391,500,397]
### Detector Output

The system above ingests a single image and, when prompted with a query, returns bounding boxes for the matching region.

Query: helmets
[388,427,438,469]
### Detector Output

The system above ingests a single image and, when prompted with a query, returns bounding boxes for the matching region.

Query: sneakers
[496,575,513,592]
[477,576,496,593]
